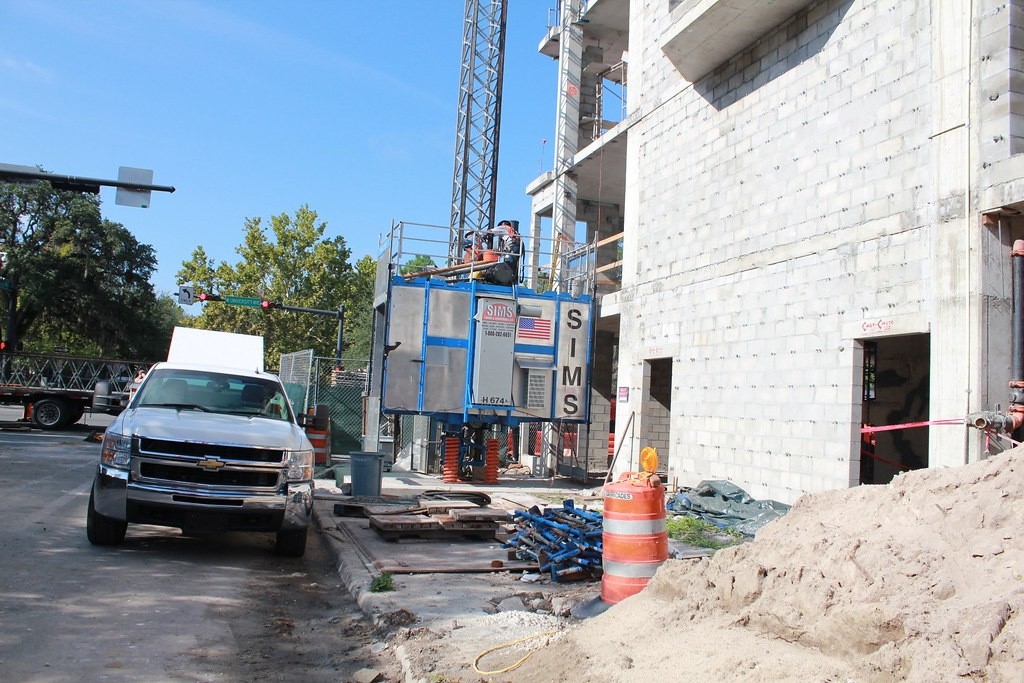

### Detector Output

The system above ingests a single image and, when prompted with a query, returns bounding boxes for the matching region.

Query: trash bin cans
[348,449,386,496]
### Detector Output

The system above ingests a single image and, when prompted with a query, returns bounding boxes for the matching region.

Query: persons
[454,231,483,278]
[482,220,524,272]
[134,369,146,385]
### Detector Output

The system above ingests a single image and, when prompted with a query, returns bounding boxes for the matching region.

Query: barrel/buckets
[600,469,669,606]
[350,450,385,497]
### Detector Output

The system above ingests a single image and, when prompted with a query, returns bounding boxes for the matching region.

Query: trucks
[0,349,151,429]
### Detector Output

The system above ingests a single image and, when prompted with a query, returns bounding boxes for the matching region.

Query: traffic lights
[262,300,282,309]
[199,293,222,302]
[51,178,100,195]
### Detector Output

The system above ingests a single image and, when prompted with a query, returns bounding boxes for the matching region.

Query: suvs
[86,363,330,558]
